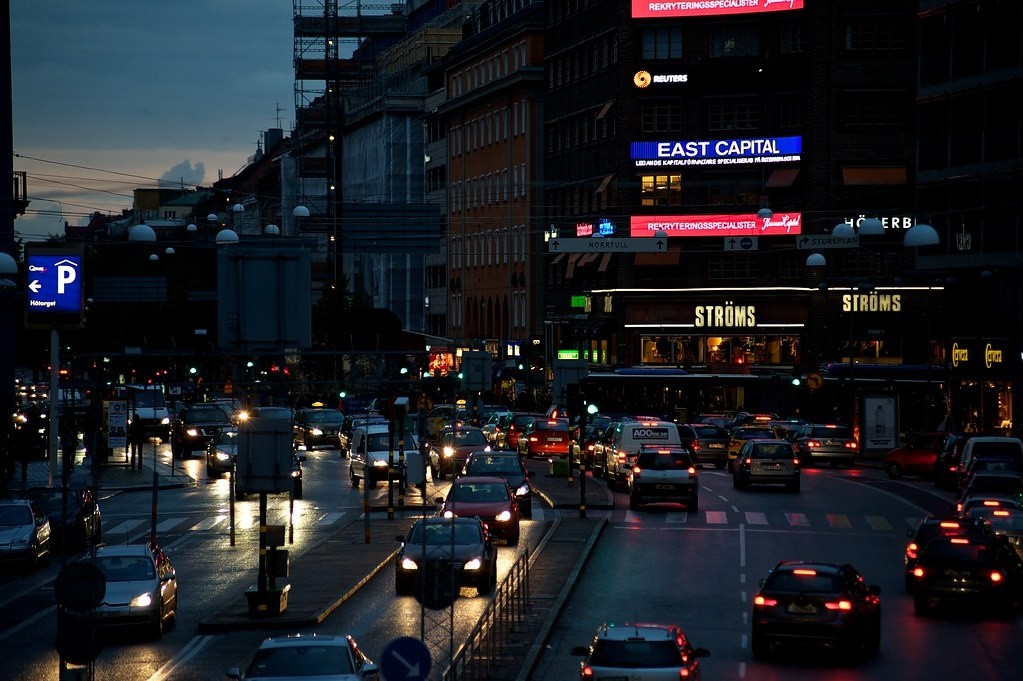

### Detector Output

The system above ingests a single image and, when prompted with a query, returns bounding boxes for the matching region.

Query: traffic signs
[795,233,860,250]
[548,235,666,252]
[723,236,757,250]
[22,242,85,320]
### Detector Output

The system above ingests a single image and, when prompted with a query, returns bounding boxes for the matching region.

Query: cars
[902,429,1023,618]
[751,558,884,659]
[234,450,307,501]
[730,437,802,494]
[57,380,664,490]
[436,474,525,545]
[882,430,964,482]
[226,632,379,681]
[0,496,53,576]
[206,425,239,479]
[790,421,860,470]
[72,541,179,639]
[25,485,102,550]
[694,408,813,440]
[570,621,711,681]
[394,515,500,596]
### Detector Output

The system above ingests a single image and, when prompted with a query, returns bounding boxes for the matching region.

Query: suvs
[601,415,684,493]
[457,451,537,517]
[676,422,731,469]
[622,443,704,513]
